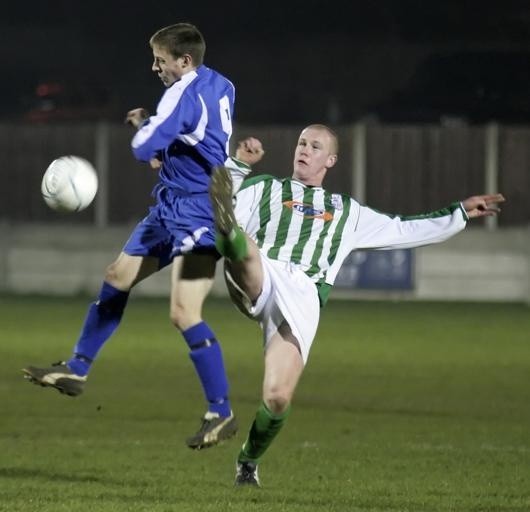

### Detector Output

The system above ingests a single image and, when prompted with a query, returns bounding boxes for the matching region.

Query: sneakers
[207,161,236,240]
[184,407,239,450]
[18,358,89,397]
[233,454,260,490]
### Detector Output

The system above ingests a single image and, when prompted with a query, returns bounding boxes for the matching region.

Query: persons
[21,21,241,449]
[206,122,507,491]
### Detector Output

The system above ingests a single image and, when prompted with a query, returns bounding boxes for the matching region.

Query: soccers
[41,155,99,214]
